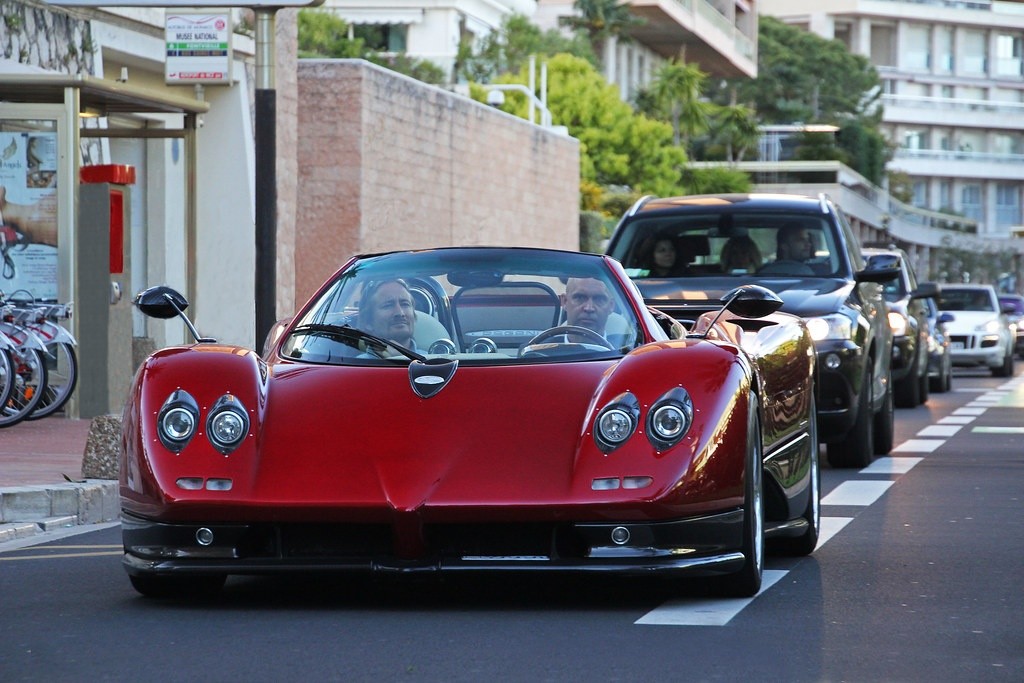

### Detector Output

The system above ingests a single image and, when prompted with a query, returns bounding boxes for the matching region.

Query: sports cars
[120,250,825,601]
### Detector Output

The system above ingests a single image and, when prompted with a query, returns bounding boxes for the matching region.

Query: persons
[637,233,691,278]
[758,222,831,276]
[539,277,633,350]
[356,278,427,359]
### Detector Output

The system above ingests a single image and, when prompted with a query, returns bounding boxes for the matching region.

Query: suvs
[938,284,1014,377]
[860,248,940,408]
[605,192,896,469]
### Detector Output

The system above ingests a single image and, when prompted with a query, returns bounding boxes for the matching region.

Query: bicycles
[0,313,48,429]
[14,308,78,418]
[0,333,13,413]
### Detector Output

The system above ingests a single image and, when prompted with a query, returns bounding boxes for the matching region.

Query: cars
[999,294,1024,357]
[922,298,955,392]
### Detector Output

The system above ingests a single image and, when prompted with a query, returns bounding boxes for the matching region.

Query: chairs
[776,234,831,275]
[674,234,722,274]
[359,310,451,353]
[555,310,632,336]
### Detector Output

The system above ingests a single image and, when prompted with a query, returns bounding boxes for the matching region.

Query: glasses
[363,278,408,300]
